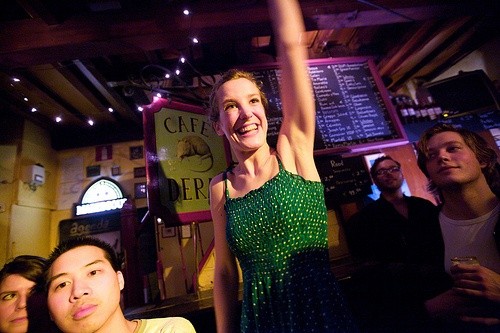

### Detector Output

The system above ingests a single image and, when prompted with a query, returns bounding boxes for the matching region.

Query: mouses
[177,134,214,173]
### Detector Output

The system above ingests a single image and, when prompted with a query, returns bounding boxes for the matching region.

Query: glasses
[376,167,399,175]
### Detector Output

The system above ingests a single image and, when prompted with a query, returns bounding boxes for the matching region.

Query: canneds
[448,256,480,304]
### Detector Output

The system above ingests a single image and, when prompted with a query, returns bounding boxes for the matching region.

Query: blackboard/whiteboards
[231,55,410,158]
[315,154,374,211]
[423,68,496,119]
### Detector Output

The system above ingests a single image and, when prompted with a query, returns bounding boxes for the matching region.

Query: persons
[407,123,500,333]
[347,155,437,333]
[42,236,198,333]
[0,255,48,333]
[208,0,359,333]
